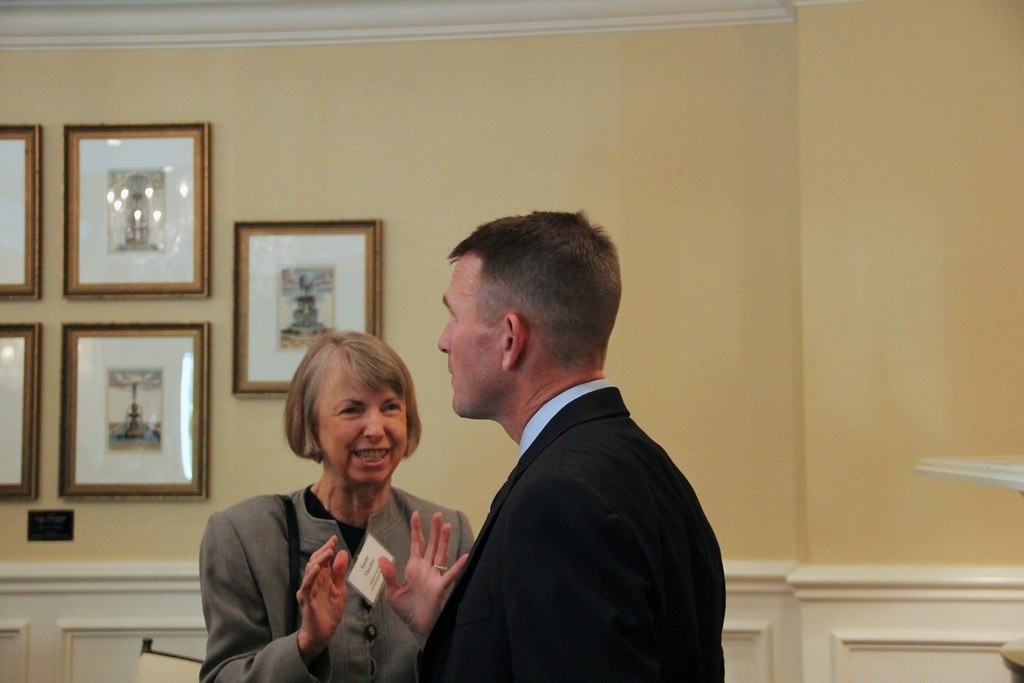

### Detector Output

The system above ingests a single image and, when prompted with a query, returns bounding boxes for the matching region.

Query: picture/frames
[63,121,209,301]
[0,322,42,500]
[0,122,42,299]
[232,219,382,394]
[59,321,210,500]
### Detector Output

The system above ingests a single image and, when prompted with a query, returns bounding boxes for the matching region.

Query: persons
[199,330,473,683]
[412,207,727,683]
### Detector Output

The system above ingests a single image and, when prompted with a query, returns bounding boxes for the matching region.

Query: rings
[434,563,446,570]
